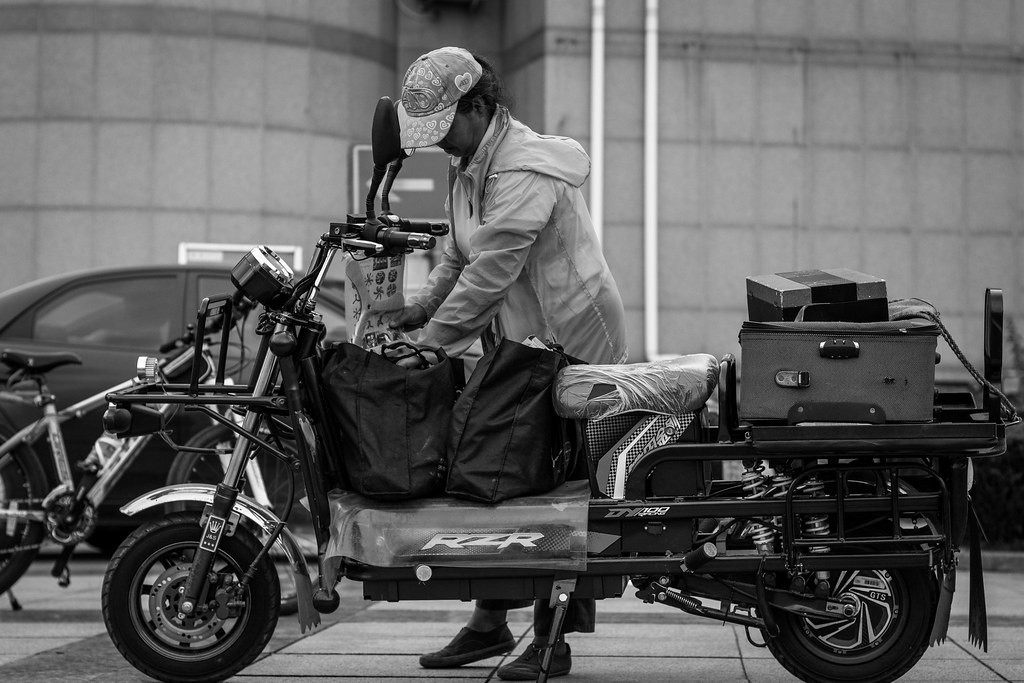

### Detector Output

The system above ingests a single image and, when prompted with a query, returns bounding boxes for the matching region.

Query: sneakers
[419,622,517,668]
[496,642,572,680]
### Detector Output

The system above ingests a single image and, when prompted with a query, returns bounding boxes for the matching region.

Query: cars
[0,264,489,560]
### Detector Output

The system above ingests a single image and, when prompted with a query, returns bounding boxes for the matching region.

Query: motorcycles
[98,94,1024,683]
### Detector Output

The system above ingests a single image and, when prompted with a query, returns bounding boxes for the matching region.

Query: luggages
[737,319,944,423]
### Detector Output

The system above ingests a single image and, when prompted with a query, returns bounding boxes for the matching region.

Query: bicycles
[0,295,339,616]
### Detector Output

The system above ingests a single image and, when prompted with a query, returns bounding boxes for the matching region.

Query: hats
[399,47,482,149]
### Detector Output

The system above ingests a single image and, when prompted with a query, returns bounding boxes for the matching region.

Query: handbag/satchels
[319,340,466,499]
[444,333,590,504]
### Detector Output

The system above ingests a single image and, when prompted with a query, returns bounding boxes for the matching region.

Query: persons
[390,46,629,680]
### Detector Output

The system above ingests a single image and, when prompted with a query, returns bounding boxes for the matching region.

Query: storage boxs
[746,263,889,327]
